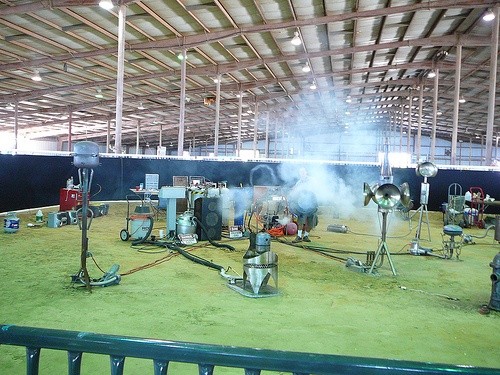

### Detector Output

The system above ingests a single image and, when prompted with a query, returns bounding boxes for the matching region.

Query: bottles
[136,185,139,191]
[36,209,43,222]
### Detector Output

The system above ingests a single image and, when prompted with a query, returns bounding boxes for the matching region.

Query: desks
[466,199,500,213]
[130,188,161,221]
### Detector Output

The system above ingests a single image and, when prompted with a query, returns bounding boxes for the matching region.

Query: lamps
[137,101,145,111]
[291,28,302,47]
[402,128,407,135]
[345,96,352,103]
[178,52,188,60]
[213,77,222,83]
[426,70,436,78]
[98,0,114,10]
[459,94,465,103]
[343,109,350,115]
[95,87,104,98]
[482,7,496,22]
[301,61,311,73]
[309,80,317,90]
[31,65,42,81]
[437,110,443,117]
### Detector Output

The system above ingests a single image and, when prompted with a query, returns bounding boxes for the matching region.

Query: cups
[151,236,155,241]
[159,230,163,237]
[139,183,143,191]
